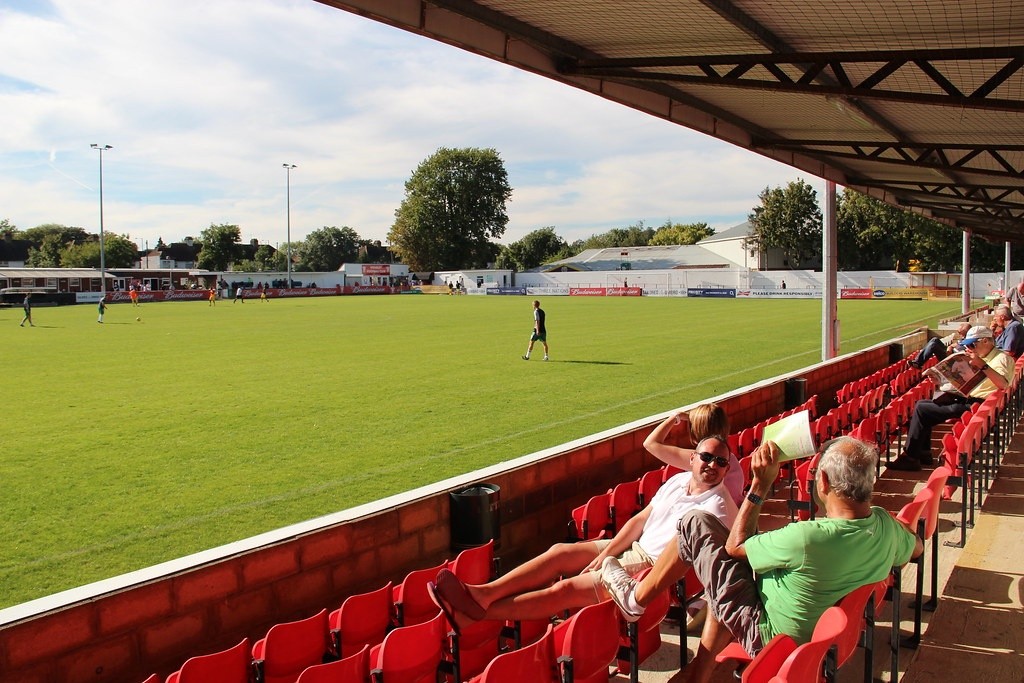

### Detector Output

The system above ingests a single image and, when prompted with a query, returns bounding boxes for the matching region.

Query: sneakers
[920,449,934,465]
[884,452,921,471]
[906,360,922,371]
[601,556,646,622]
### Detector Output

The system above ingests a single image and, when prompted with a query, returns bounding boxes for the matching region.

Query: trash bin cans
[785,377,808,410]
[450,483,500,552]
[888,343,903,364]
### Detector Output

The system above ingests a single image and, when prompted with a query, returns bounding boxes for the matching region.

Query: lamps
[928,166,956,184]
[824,93,876,129]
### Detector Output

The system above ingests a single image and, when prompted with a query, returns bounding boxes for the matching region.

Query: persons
[449,281,453,295]
[264,281,269,289]
[129,280,151,291]
[521,300,548,361]
[952,355,975,382]
[234,285,244,303]
[97,295,108,323]
[642,404,744,509]
[114,282,119,291]
[261,285,269,303]
[600,435,924,683]
[456,280,463,295]
[20,293,34,327]
[208,286,216,306]
[161,284,166,290]
[130,288,138,306]
[781,280,786,289]
[427,435,740,639]
[624,277,628,288]
[883,281,1024,471]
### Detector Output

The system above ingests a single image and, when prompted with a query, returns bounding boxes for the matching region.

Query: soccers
[137,304,140,307]
[136,317,141,321]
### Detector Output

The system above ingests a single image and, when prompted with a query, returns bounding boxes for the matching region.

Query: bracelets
[747,492,763,506]
[981,363,989,370]
[674,414,681,426]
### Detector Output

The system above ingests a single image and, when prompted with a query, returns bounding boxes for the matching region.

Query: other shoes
[521,355,529,360]
[98,321,103,323]
[30,324,34,327]
[542,356,548,361]
[20,324,24,327]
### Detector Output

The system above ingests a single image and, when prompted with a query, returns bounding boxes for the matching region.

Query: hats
[1018,282,1024,297]
[960,325,992,346]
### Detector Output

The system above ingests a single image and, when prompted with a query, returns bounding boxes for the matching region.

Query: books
[762,409,816,463]
[921,352,988,398]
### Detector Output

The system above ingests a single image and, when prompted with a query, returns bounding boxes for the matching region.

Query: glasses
[966,339,983,350]
[809,467,820,475]
[694,451,730,467]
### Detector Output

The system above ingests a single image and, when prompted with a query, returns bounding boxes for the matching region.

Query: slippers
[436,568,486,621]
[427,581,464,636]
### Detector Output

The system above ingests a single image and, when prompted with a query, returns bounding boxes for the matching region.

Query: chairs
[143,333,1024,683]
